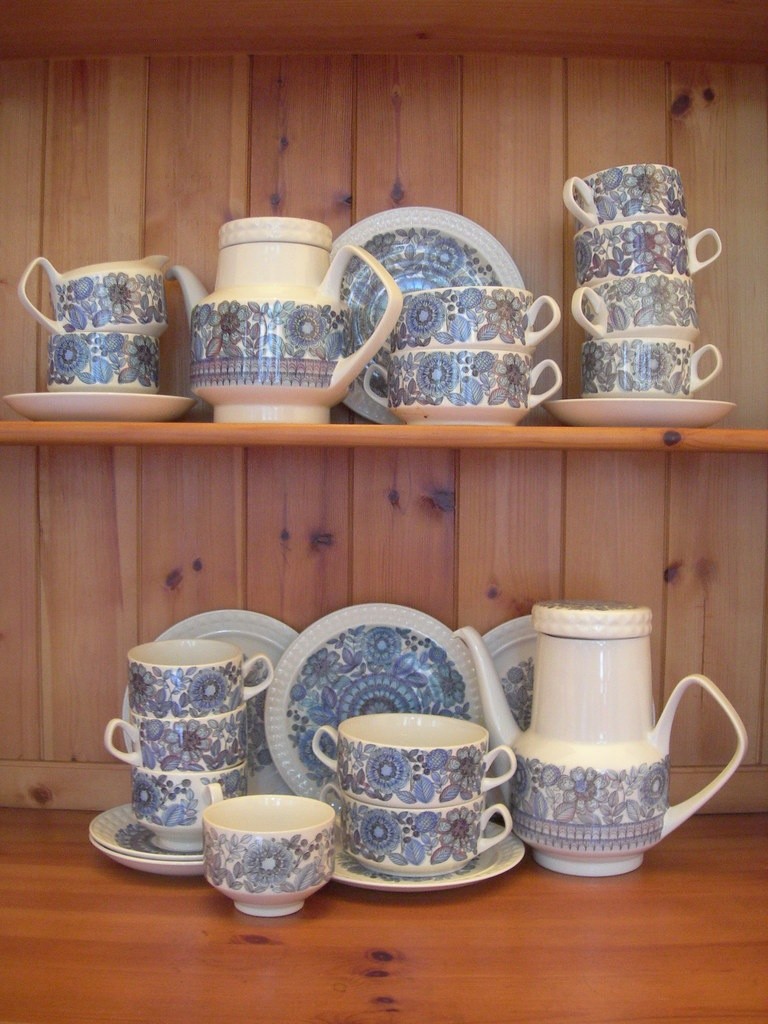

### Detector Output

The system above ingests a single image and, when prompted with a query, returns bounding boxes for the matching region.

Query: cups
[311,713,518,808]
[561,163,723,398]
[318,784,514,878]
[104,641,274,854]
[363,349,563,426]
[394,286,561,351]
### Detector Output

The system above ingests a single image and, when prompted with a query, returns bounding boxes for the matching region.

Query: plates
[3,391,195,421]
[90,804,203,877]
[122,608,299,794]
[328,821,525,892]
[331,207,525,424]
[265,604,481,796]
[541,397,736,426]
[482,614,533,731]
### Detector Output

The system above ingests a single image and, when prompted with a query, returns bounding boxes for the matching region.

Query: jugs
[172,217,402,424]
[453,601,748,878]
[18,256,172,338]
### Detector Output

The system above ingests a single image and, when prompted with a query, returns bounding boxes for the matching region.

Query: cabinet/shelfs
[0,0,768,1024]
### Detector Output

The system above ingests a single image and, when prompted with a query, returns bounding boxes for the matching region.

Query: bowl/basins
[44,331,160,393]
[203,795,336,916]
